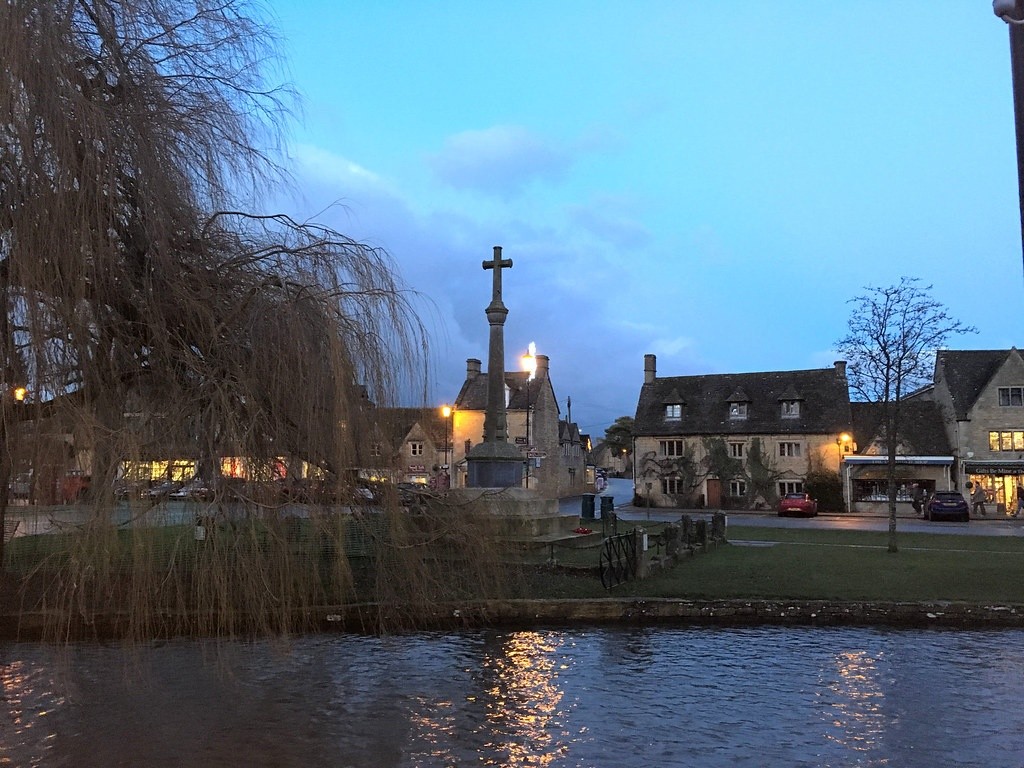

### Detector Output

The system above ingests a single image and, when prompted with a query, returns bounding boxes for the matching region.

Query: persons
[908,482,922,515]
[970,481,987,516]
[1010,482,1024,518]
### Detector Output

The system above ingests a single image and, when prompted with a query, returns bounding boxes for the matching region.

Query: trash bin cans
[581,493,595,518]
[600,496,614,518]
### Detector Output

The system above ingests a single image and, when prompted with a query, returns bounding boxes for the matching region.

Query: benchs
[3,519,20,543]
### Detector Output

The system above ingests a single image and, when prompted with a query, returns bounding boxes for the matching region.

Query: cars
[923,490,970,523]
[12,460,433,505]
[779,493,818,519]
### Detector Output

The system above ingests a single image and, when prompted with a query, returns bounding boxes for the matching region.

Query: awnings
[844,458,954,464]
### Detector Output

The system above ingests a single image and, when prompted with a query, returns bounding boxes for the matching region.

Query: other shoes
[1011,515,1017,518]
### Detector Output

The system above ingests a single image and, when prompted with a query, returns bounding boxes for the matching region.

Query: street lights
[441,402,449,465]
[519,349,535,488]
